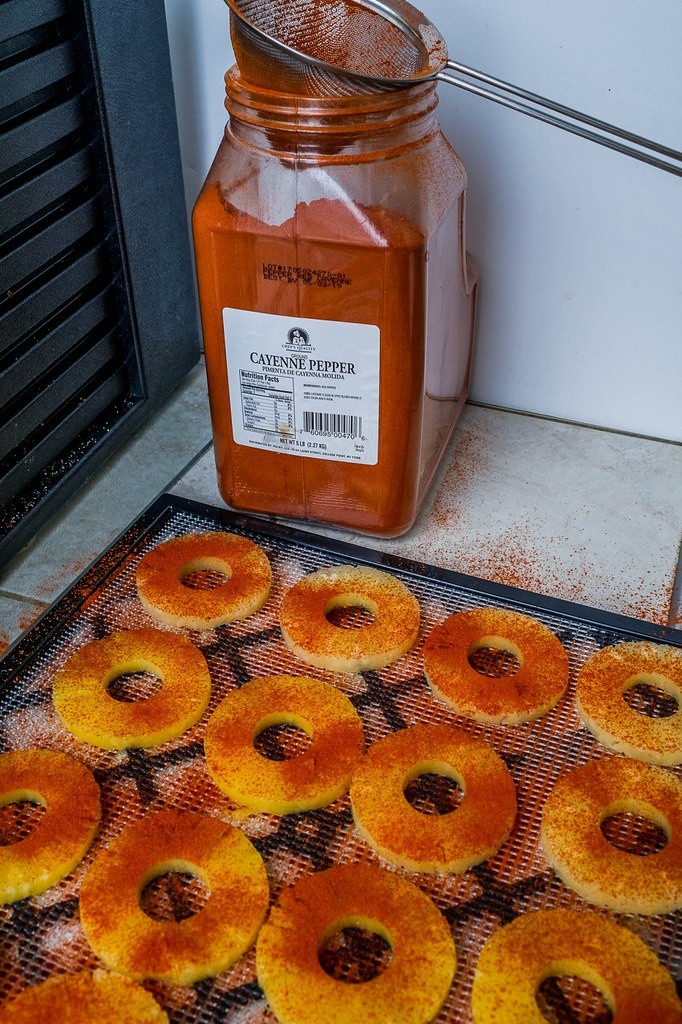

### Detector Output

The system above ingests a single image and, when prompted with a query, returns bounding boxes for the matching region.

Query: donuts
[0,534,681,1024]
